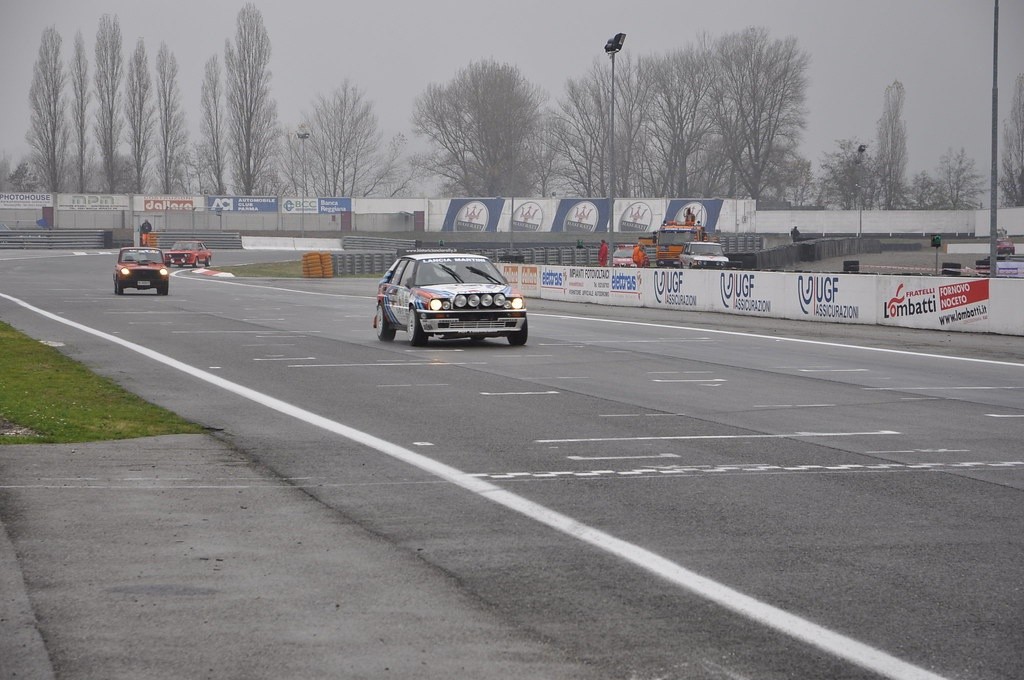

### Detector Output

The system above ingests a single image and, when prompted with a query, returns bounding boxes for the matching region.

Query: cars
[113,248,170,296]
[373,252,529,347]
[678,242,730,270]
[998,239,1016,255]
[163,241,211,267]
[608,243,639,267]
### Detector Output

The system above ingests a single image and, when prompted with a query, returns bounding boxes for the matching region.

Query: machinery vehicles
[654,209,719,267]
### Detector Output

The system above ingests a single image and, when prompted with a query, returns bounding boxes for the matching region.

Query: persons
[598,239,608,267]
[633,242,650,268]
[792,226,800,242]
[142,218,152,232]
[685,209,695,226]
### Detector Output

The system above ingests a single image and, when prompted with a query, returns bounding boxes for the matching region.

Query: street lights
[298,132,310,238]
[857,144,866,238]
[605,33,627,271]
